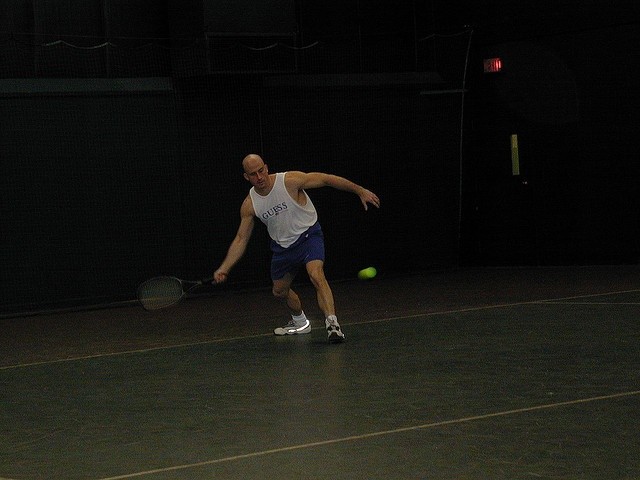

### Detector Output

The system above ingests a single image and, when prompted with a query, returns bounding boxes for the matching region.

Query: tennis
[359,267,377,280]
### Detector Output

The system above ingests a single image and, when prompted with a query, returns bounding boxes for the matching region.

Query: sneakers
[326,320,346,344]
[273,319,311,336]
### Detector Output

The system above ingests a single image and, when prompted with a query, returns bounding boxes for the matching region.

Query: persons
[212,154,380,342]
